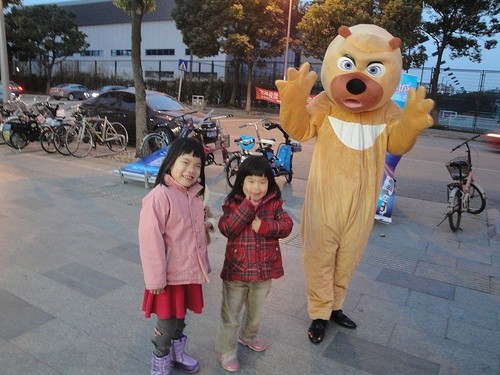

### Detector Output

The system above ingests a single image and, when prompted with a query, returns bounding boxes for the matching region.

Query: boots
[169,333,200,374]
[151,351,170,375]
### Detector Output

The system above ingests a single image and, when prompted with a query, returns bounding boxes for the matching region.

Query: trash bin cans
[192,95,205,109]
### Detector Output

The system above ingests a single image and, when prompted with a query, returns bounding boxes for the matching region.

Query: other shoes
[238,335,267,351]
[217,350,240,372]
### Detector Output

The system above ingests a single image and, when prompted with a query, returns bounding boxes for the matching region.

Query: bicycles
[0,94,128,158]
[435,132,488,234]
[137,106,233,163]
[226,117,268,189]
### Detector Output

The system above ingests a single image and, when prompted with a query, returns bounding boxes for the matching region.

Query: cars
[0,80,22,96]
[76,87,219,148]
[49,83,90,101]
[85,84,126,101]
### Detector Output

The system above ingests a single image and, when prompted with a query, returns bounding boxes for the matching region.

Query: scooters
[239,121,303,184]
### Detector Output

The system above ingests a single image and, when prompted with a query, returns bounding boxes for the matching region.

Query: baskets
[214,134,230,149]
[445,155,469,180]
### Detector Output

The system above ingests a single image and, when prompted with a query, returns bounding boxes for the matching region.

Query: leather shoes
[330,309,357,329]
[308,318,328,344]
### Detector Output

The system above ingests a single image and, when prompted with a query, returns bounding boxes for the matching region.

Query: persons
[217,156,293,373]
[195,177,216,275]
[139,137,212,375]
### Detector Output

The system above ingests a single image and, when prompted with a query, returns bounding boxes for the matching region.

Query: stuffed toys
[275,23,435,344]
